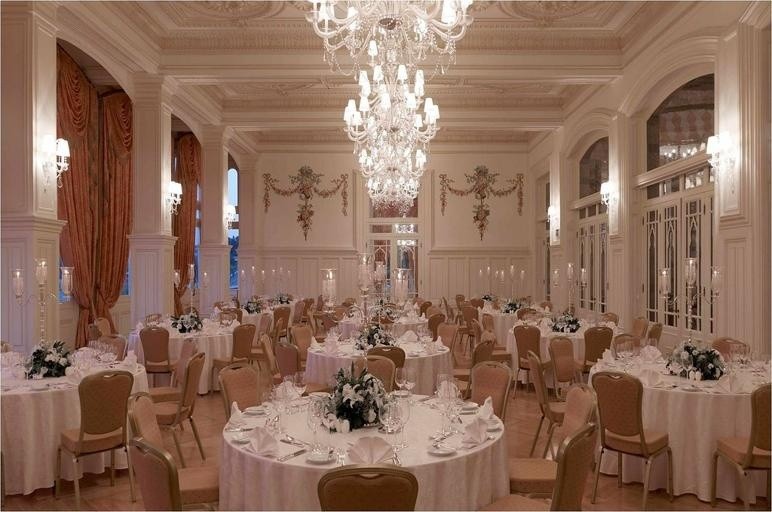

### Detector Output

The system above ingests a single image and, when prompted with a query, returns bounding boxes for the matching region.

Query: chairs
[711,383,771,510]
[505,382,596,502]
[126,392,221,509]
[53,370,136,509]
[591,371,676,511]
[127,353,207,467]
[86,296,663,398]
[479,422,597,509]
[130,435,184,509]
[467,360,514,426]
[711,336,750,360]
[217,363,262,426]
[525,349,567,461]
[317,464,419,510]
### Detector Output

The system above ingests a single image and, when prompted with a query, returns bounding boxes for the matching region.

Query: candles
[36,259,47,283]
[60,266,74,293]
[394,273,408,300]
[240,266,265,282]
[553,263,588,288]
[708,265,723,293]
[323,272,335,303]
[478,265,524,281]
[660,268,672,295]
[684,257,697,286]
[174,264,209,289]
[12,269,24,294]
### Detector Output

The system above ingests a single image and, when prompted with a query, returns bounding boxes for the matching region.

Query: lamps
[705,135,734,170]
[167,181,184,219]
[44,138,72,187]
[600,182,615,205]
[301,0,475,214]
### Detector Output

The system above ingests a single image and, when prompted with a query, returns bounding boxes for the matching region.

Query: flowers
[23,339,73,378]
[666,338,724,381]
[329,363,386,423]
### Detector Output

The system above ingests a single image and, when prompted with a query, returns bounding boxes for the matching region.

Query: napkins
[461,416,492,449]
[246,427,279,457]
[347,436,396,466]
[476,395,497,424]
[434,381,462,401]
[226,401,247,428]
[269,380,307,406]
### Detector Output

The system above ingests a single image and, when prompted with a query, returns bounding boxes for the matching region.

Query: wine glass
[225,367,503,465]
[592,339,768,396]
[0,332,139,391]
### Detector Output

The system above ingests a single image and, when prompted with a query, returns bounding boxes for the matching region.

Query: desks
[590,351,770,504]
[3,352,166,499]
[217,389,510,509]
